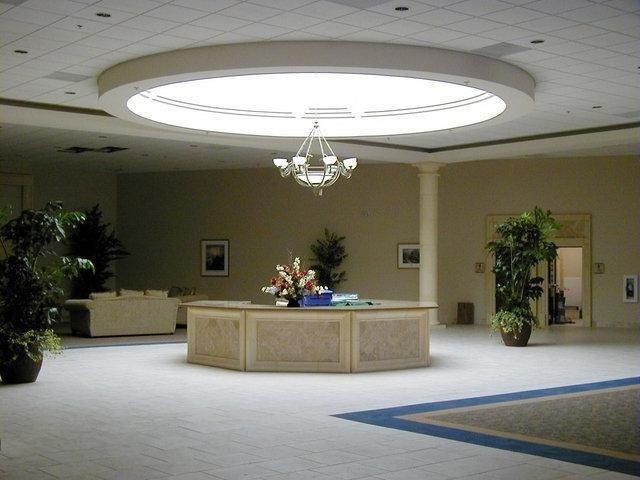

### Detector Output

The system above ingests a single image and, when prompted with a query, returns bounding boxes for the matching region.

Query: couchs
[62,289,181,336]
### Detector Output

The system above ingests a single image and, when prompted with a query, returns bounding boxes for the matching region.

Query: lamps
[272,118,358,197]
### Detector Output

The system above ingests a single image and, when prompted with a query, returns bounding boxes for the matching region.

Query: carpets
[327,377,639,480]
[52,319,188,350]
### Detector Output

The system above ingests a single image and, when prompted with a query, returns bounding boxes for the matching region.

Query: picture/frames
[397,242,421,269]
[200,238,230,277]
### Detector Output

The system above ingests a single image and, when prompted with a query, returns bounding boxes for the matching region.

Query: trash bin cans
[457,302,474,324]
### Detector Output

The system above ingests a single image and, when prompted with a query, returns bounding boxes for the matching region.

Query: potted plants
[0,200,96,385]
[484,206,559,348]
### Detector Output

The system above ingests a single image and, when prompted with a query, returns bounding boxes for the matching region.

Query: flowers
[261,256,330,298]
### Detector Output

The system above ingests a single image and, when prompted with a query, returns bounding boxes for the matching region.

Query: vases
[286,296,301,308]
